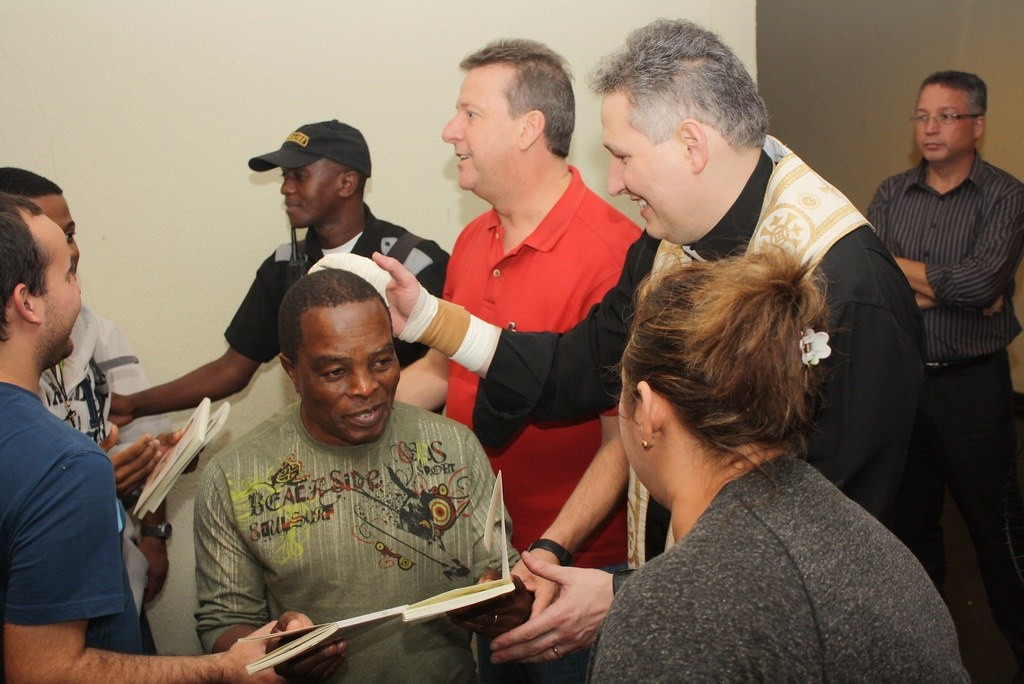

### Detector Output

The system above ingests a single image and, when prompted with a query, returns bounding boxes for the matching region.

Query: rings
[552,646,563,659]
[493,615,497,627]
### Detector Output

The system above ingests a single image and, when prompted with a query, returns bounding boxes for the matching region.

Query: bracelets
[528,539,573,567]
[612,569,636,596]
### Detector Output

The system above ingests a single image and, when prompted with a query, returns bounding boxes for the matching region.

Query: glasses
[909,113,980,126]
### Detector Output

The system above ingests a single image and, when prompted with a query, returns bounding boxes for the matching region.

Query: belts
[924,355,996,377]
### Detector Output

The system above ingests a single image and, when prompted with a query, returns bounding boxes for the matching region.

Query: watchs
[141,522,172,538]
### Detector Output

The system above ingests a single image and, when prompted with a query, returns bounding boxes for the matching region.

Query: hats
[251,119,371,176]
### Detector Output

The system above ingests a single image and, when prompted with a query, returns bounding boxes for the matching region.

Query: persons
[0,193,286,684]
[82,304,169,656]
[107,119,450,415]
[305,20,929,665]
[394,41,644,684]
[865,71,1024,684]
[193,270,533,684]
[0,166,206,618]
[584,245,972,684]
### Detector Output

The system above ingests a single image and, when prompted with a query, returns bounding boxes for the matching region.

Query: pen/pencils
[508,321,515,331]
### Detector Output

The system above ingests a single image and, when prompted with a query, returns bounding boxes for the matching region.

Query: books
[237,470,516,676]
[132,396,230,519]
